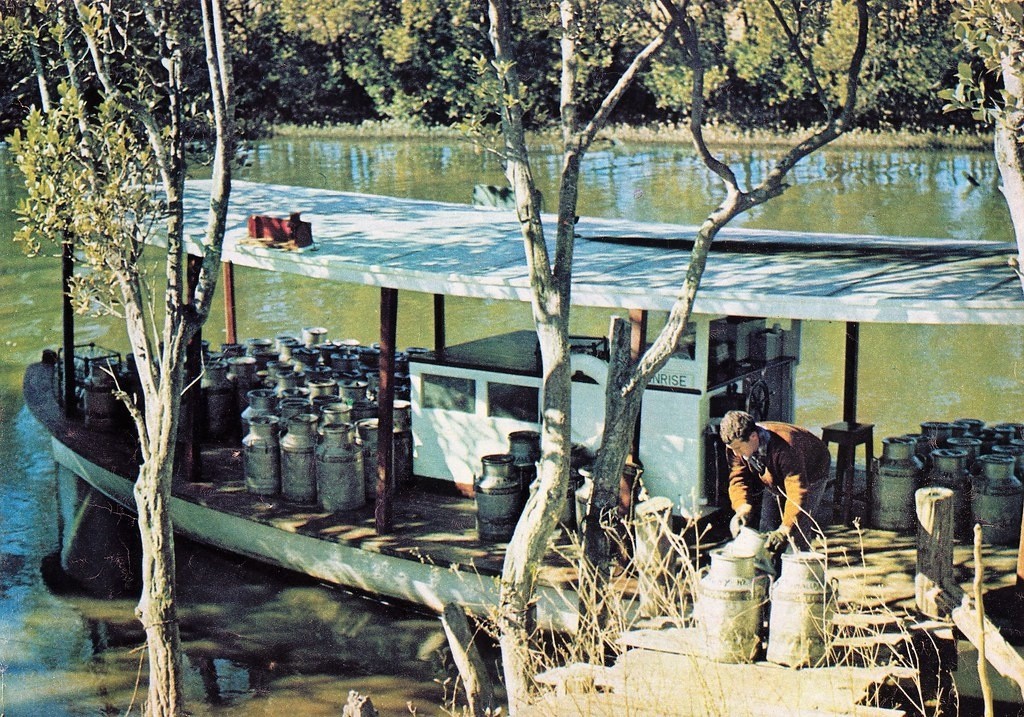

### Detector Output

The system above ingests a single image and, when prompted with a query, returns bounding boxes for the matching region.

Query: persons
[720,410,831,623]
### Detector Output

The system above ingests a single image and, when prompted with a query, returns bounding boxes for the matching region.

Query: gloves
[729,513,747,540]
[759,530,786,551]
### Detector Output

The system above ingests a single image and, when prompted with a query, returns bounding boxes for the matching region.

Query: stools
[818,421,875,519]
